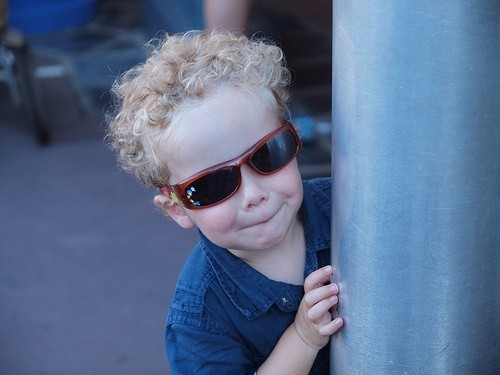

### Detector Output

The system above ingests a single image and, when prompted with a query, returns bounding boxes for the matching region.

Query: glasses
[159,119,303,211]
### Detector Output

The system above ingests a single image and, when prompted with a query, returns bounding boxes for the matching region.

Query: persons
[97,27,346,374]
